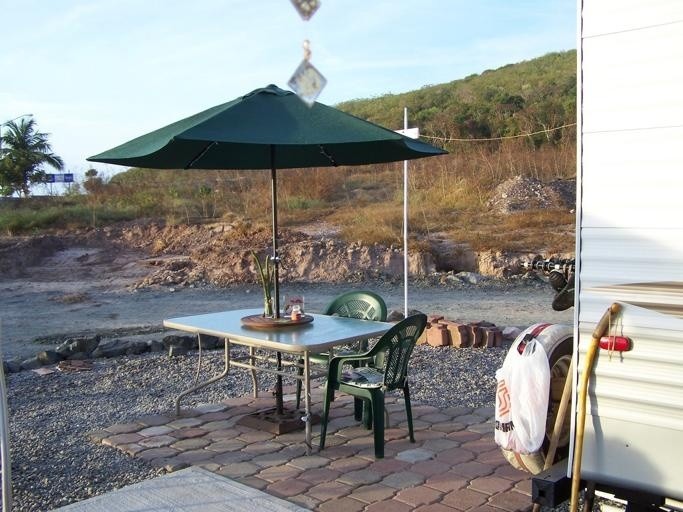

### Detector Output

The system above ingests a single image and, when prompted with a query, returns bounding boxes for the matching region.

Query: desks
[163,307,401,457]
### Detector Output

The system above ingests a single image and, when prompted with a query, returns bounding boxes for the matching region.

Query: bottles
[290,305,301,321]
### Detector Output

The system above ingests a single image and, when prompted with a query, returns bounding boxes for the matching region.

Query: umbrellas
[85,83,449,417]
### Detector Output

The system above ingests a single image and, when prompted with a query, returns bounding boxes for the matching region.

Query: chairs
[295,290,427,459]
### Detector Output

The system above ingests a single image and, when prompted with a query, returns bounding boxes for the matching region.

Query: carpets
[46,464,317,511]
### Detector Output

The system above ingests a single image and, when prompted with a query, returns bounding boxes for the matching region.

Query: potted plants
[250,248,278,316]
